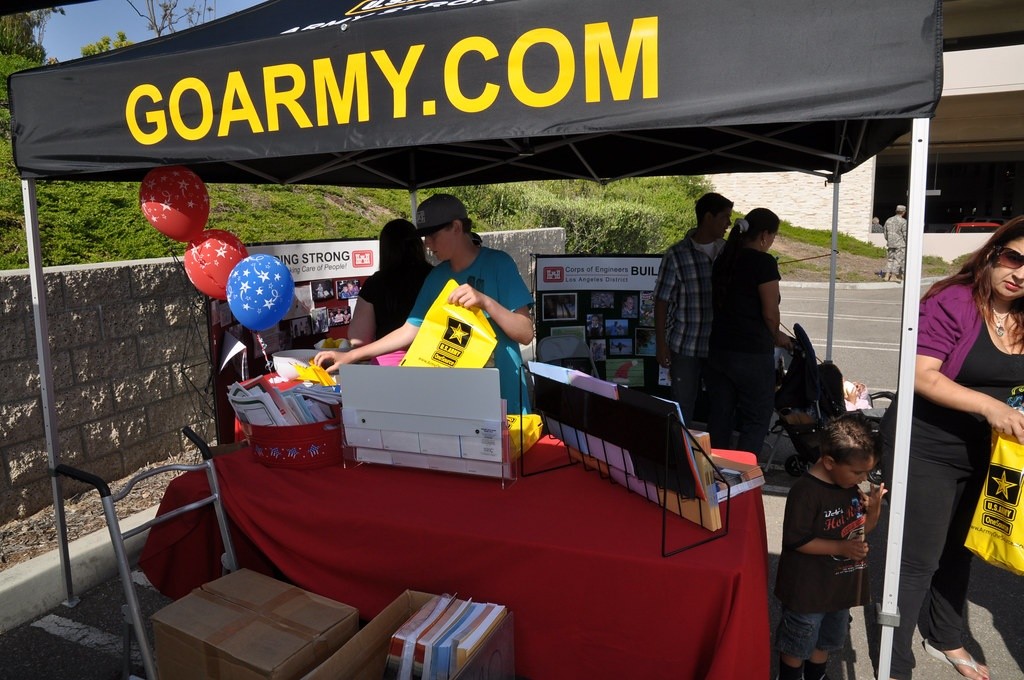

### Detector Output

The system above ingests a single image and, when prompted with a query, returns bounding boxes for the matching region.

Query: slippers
[924,639,979,680]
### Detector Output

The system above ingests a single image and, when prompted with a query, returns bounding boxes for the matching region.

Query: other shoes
[883,272,900,283]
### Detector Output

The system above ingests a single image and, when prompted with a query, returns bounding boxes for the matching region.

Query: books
[380,592,508,680]
[227,363,345,427]
[527,360,766,532]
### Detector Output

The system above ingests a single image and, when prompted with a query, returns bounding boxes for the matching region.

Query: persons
[313,192,536,415]
[773,410,889,680]
[842,377,874,412]
[706,207,796,453]
[346,218,436,367]
[883,205,908,283]
[875,214,1024,680]
[652,192,734,430]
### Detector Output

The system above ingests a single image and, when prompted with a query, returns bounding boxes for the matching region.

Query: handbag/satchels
[965,407,1024,576]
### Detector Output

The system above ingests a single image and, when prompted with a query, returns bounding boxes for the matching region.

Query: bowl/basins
[272,349,318,379]
[314,339,355,352]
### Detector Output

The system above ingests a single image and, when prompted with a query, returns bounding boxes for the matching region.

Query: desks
[138,443,769,680]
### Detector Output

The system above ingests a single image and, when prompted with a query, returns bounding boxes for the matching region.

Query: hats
[410,194,467,236]
[896,205,906,211]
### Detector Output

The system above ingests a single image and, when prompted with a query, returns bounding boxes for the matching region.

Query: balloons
[139,164,296,389]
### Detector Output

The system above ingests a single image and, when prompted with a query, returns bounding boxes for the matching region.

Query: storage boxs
[147,566,359,680]
[301,588,515,680]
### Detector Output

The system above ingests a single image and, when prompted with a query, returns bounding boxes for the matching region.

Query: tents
[7,0,948,679]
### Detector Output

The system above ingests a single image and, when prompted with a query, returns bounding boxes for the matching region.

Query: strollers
[760,322,895,478]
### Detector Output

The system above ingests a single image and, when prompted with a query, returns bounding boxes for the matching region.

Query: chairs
[534,335,600,379]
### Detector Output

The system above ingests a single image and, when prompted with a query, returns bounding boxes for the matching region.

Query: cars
[946,216,1006,233]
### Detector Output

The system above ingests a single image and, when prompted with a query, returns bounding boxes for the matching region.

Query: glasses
[992,241,1024,270]
